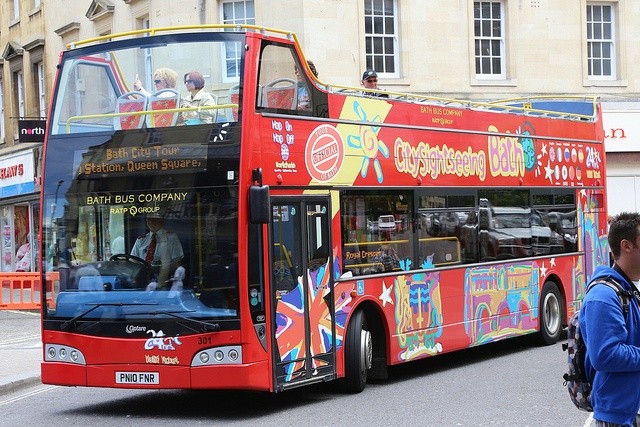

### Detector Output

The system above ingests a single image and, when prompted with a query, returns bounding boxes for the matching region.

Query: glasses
[154,80,161,83]
[184,79,193,84]
[363,79,378,83]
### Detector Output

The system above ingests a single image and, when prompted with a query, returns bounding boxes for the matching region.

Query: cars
[368,222,378,233]
[456,212,468,221]
[431,210,461,237]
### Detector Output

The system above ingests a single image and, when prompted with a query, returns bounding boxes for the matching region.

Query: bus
[39,26,608,395]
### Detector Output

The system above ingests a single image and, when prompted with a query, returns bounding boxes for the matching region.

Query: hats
[363,70,378,80]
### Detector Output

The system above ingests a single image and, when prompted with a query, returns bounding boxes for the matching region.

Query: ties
[146,233,157,262]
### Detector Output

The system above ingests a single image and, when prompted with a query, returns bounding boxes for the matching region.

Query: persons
[293,58,327,111]
[174,70,216,125]
[360,71,388,98]
[582,212,639,427]
[132,68,177,99]
[127,203,186,290]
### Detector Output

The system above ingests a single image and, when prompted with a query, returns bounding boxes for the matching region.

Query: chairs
[225,84,261,126]
[337,89,361,93]
[420,100,444,104]
[113,92,146,130]
[395,97,416,101]
[210,95,218,123]
[146,89,181,128]
[162,217,195,290]
[261,79,298,110]
[445,102,464,105]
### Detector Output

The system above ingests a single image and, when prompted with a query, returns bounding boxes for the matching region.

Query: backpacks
[560,274,634,412]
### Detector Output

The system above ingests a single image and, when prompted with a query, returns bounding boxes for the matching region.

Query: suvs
[464,196,565,262]
[378,215,396,233]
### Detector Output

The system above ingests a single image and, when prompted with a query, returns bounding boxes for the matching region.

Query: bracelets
[137,86,143,91]
